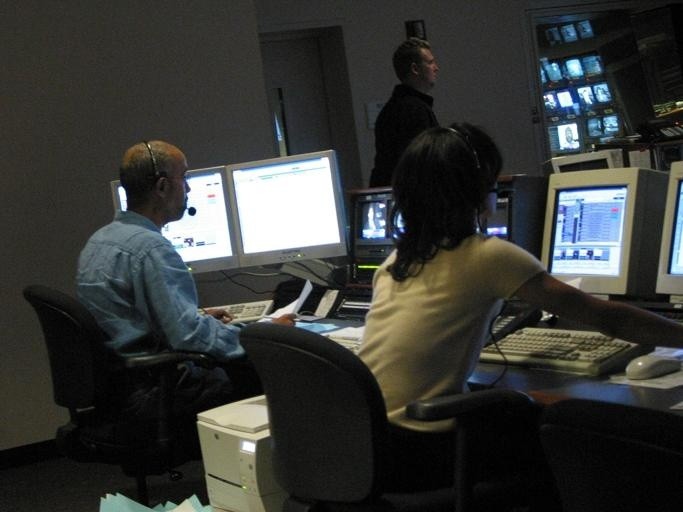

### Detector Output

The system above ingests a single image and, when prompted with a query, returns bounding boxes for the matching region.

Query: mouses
[624,353,681,380]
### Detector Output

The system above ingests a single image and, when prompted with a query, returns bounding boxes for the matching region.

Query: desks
[250,315,683,413]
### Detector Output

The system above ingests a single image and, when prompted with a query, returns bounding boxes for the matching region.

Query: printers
[194,393,289,511]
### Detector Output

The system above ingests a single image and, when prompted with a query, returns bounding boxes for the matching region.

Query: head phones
[142,139,169,199]
[441,122,497,204]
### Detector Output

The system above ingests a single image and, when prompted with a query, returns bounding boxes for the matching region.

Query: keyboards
[317,332,364,359]
[479,326,640,378]
[197,298,274,322]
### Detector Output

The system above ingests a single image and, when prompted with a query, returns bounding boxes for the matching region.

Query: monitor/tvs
[109,164,240,276]
[477,172,547,254]
[654,160,683,311]
[226,149,351,270]
[345,185,407,267]
[539,167,668,301]
[531,8,656,169]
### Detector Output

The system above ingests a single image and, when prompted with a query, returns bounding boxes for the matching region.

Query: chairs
[237,323,681,512]
[21,285,241,507]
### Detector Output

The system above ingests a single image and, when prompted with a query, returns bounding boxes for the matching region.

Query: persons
[367,36,440,187]
[358,119,682,493]
[74,139,298,433]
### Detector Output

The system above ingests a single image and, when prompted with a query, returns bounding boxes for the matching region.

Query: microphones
[186,206,197,217]
